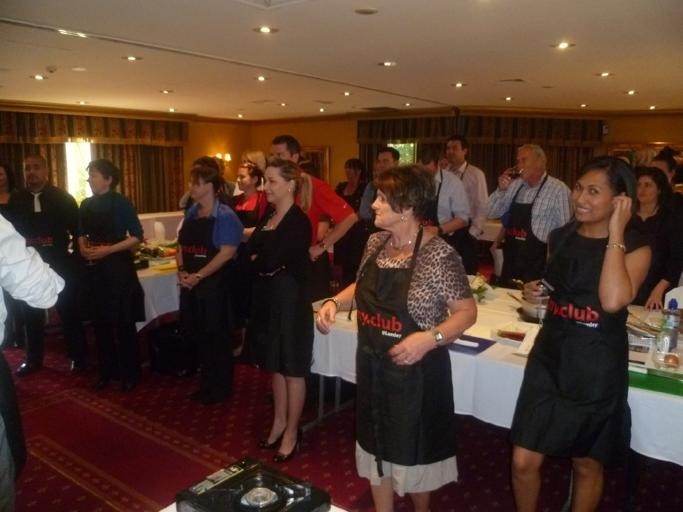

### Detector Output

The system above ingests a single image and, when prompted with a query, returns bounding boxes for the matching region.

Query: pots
[505,278,548,319]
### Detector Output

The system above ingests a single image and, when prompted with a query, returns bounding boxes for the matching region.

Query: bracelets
[321,299,341,313]
[605,243,626,251]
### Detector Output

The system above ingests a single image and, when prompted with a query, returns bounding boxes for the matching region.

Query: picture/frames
[298,145,331,186]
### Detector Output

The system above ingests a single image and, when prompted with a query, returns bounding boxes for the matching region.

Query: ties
[31,192,43,213]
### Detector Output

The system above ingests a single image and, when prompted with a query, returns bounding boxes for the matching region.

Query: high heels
[258,436,280,449]
[273,442,297,463]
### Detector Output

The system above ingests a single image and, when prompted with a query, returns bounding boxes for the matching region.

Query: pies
[626,306,664,337]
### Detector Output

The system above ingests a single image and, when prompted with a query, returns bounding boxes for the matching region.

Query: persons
[486,144,571,290]
[509,157,651,512]
[1,154,83,373]
[316,166,477,512]
[615,147,683,310]
[74,159,146,391]
[176,133,358,463]
[0,215,65,512]
[363,140,489,274]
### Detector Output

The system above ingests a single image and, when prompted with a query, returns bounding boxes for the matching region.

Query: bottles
[661,298,680,349]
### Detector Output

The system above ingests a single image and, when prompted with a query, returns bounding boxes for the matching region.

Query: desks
[135,210,185,243]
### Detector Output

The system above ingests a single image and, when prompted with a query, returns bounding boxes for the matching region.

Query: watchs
[431,329,443,346]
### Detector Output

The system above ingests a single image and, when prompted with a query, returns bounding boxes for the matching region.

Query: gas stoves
[173,454,332,512]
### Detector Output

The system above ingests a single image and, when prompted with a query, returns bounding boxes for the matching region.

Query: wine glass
[501,165,523,188]
[83,232,96,267]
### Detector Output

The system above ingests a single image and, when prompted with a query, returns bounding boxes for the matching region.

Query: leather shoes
[15,362,42,376]
